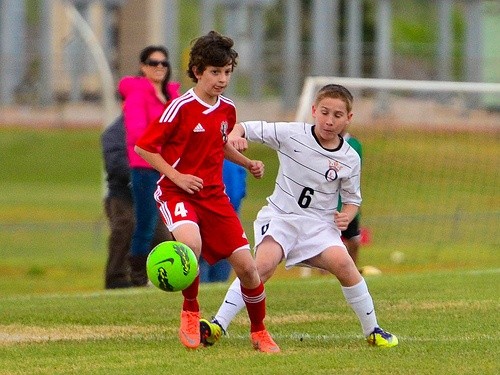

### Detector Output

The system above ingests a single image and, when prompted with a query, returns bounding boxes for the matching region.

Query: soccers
[146,240,199,293]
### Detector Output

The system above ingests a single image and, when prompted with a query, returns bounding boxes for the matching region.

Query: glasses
[144,60,169,67]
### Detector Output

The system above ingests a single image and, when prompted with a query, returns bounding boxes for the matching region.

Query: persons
[200,83,398,348]
[198,161,249,284]
[338,112,365,273]
[134,30,284,354]
[98,113,174,290]
[117,43,181,289]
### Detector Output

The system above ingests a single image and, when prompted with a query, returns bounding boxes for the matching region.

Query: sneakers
[179,304,201,349]
[200,319,223,347]
[250,329,281,354]
[367,328,398,349]
